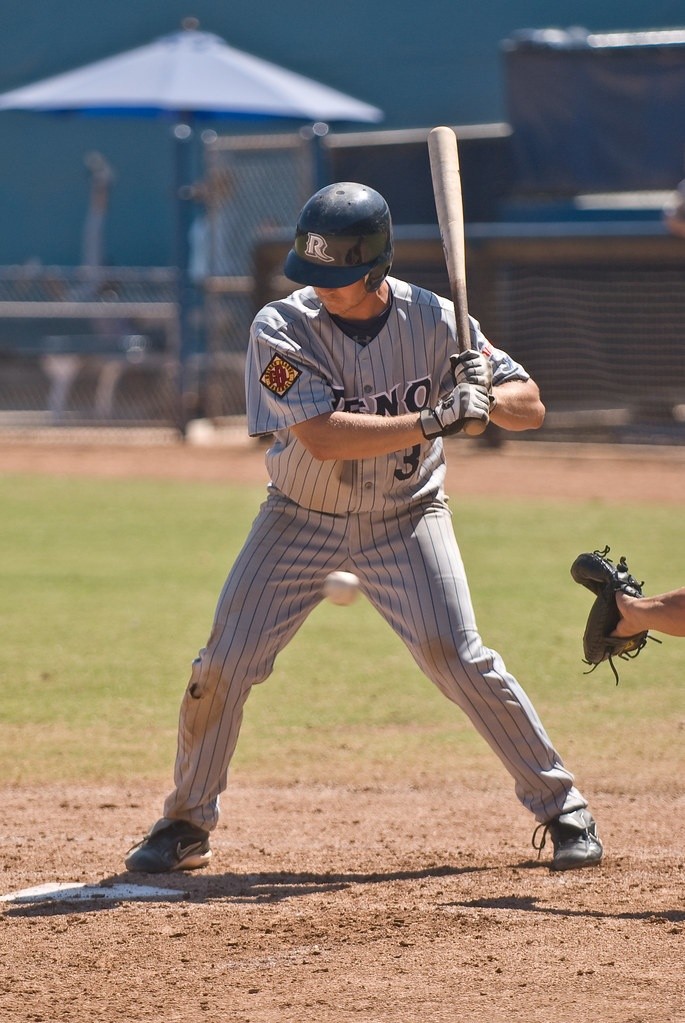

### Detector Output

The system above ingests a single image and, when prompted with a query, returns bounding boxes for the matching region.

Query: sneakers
[124,817,212,874]
[531,808,603,870]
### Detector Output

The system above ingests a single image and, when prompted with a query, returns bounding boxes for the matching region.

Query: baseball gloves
[570,552,648,663]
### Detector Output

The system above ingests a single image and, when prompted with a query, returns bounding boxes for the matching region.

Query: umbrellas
[0,18,386,421]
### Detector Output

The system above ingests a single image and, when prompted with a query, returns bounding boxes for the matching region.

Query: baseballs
[325,571,360,606]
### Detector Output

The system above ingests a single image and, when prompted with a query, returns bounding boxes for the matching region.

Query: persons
[570,552,685,665]
[9,149,143,414]
[126,180,604,876]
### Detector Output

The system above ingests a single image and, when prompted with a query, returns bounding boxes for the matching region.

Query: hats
[283,182,394,292]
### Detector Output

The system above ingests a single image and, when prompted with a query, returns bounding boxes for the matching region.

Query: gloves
[419,382,490,440]
[449,350,498,414]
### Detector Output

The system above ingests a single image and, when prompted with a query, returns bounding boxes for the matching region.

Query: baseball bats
[427,126,487,435]
